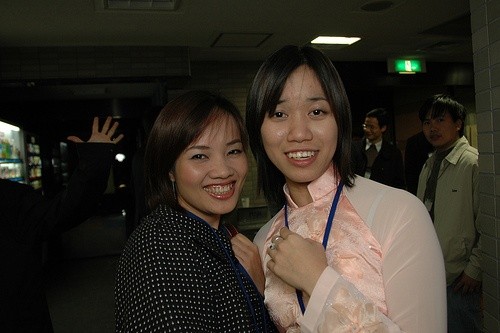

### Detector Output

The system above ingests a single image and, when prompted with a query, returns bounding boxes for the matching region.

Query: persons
[351,106,430,198]
[415,89,485,333]
[243,41,448,333]
[0,114,125,333]
[114,90,280,333]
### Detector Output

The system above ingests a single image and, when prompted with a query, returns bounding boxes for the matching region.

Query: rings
[266,244,272,253]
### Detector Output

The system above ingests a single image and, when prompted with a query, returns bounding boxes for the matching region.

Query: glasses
[361,123,380,129]
